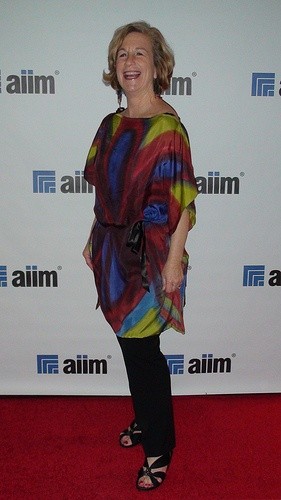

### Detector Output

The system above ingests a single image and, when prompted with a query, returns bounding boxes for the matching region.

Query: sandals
[120,420,172,490]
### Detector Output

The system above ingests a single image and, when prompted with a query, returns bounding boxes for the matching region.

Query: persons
[82,21,199,491]
